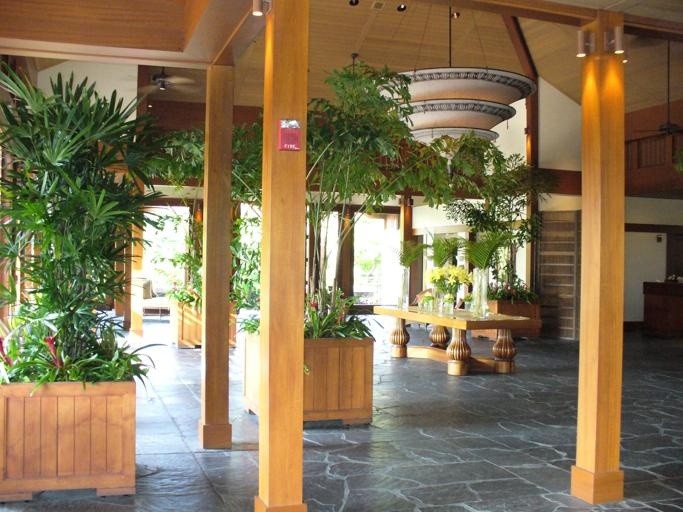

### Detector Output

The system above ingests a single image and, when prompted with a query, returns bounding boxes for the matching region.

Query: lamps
[253,0,272,16]
[577,24,629,63]
[367,5,537,179]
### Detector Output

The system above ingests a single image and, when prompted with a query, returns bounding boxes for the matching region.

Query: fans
[633,39,683,136]
